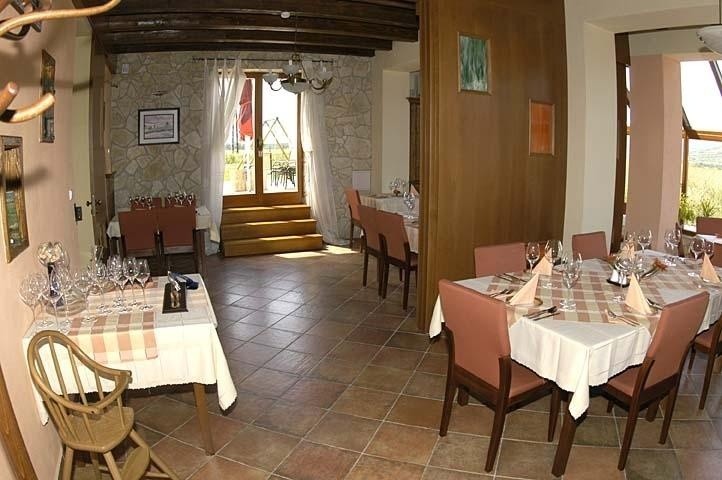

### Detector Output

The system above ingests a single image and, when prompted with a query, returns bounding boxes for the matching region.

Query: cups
[564,251,583,274]
[702,238,715,258]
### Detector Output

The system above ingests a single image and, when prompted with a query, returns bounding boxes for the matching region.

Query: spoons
[529,308,557,319]
[608,310,640,326]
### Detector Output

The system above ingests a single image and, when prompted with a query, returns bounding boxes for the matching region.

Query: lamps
[262,12,333,95]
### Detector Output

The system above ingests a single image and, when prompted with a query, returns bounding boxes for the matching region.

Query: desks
[20,274,238,455]
[106,206,221,281]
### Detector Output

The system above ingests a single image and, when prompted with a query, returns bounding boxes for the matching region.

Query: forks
[488,289,514,298]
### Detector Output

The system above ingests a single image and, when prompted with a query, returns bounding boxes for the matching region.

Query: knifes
[533,312,560,321]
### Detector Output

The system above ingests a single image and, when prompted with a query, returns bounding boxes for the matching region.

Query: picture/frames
[1,135,30,263]
[528,99,556,157]
[138,108,180,145]
[456,31,493,95]
[40,49,55,143]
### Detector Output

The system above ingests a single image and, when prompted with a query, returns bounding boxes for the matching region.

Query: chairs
[265,161,296,190]
[343,180,420,312]
[117,194,199,273]
[27,330,180,480]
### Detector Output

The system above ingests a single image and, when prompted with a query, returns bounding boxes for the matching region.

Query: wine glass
[612,228,681,298]
[687,236,702,278]
[136,258,152,312]
[123,254,141,306]
[391,176,408,197]
[75,243,107,324]
[167,191,194,208]
[560,261,582,308]
[544,240,562,287]
[126,192,155,212]
[20,267,74,332]
[402,192,417,222]
[107,254,128,311]
[114,262,129,311]
[524,240,539,282]
